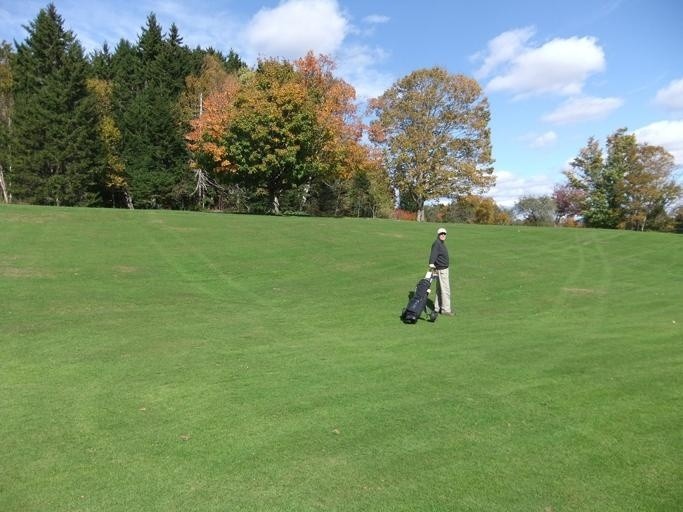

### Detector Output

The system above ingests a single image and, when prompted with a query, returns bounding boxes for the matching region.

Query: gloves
[428,264,437,269]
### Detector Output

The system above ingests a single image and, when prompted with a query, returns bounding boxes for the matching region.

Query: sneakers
[441,312,456,316]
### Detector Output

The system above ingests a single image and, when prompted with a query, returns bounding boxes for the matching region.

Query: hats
[438,228,447,234]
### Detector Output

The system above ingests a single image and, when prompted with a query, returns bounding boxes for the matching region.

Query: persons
[429,228,454,315]
